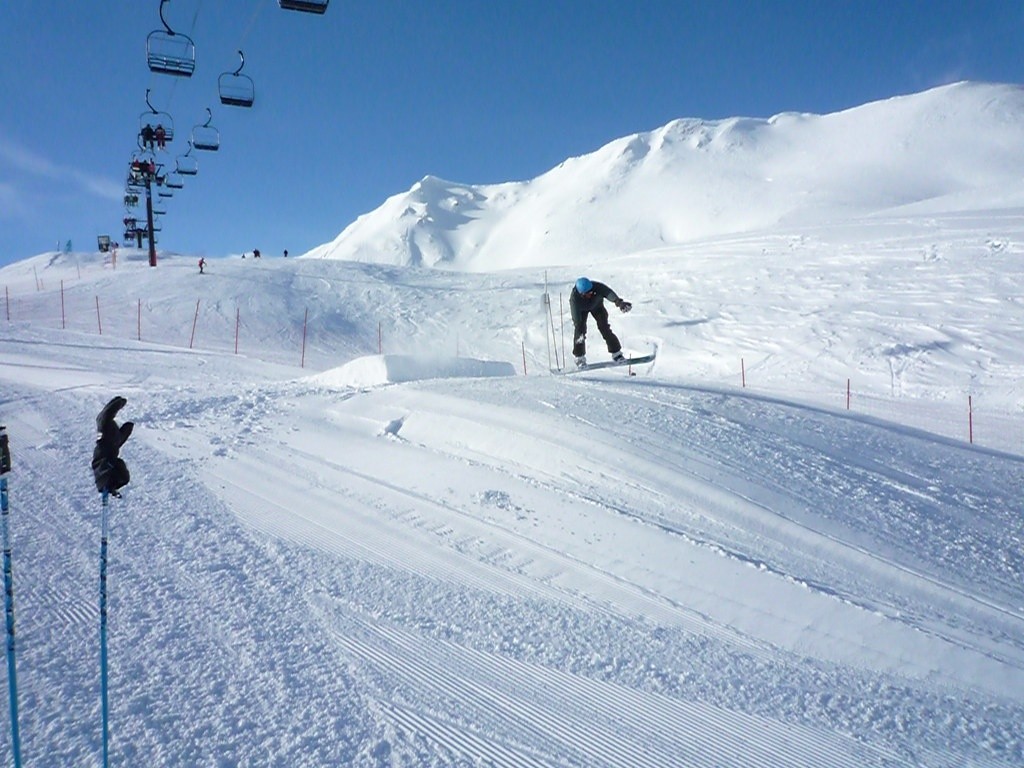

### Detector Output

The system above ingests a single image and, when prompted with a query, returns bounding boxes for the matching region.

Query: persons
[110,158,156,253]
[283,249,288,257]
[198,257,207,274]
[253,249,260,258]
[65,240,72,254]
[141,124,154,150]
[154,124,167,151]
[568,277,633,371]
[241,254,245,258]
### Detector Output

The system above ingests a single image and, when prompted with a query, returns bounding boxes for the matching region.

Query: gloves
[92,396,134,500]
[615,298,632,314]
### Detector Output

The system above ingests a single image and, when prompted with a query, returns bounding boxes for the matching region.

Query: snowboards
[550,354,656,375]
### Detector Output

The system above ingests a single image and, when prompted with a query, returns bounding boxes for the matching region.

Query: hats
[575,277,594,295]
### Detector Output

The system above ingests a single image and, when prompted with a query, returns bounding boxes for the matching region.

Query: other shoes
[612,351,627,364]
[574,356,588,370]
